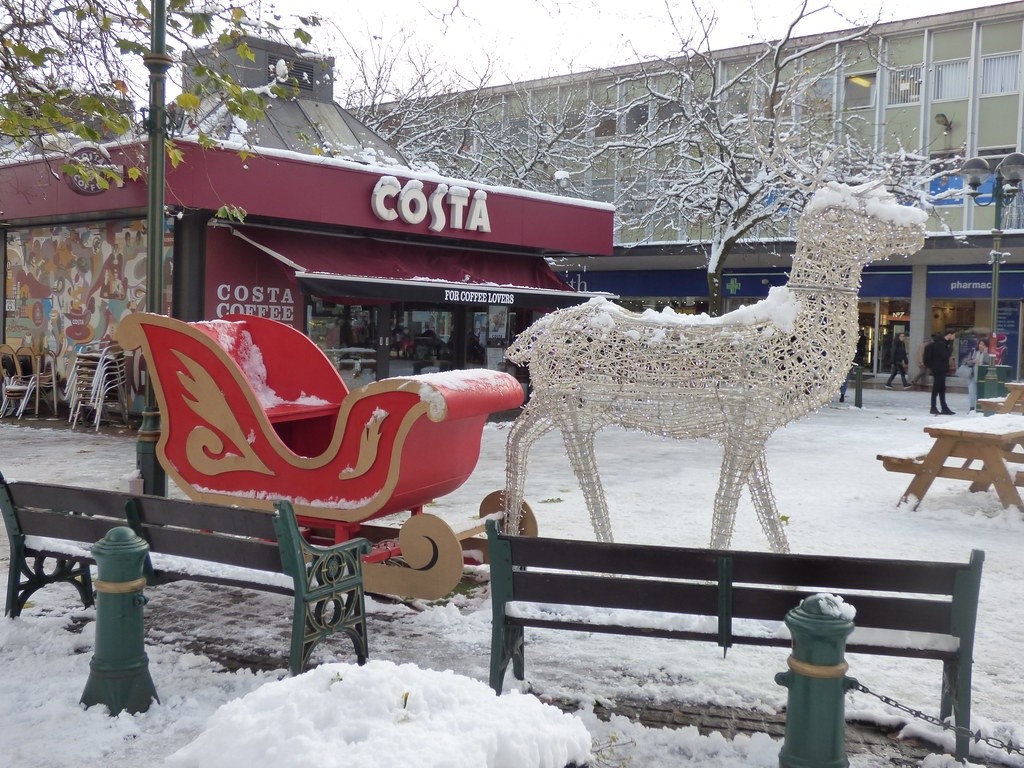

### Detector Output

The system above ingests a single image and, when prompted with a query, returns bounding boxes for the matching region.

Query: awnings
[229,224,618,300]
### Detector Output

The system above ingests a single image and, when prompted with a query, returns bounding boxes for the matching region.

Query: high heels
[840,394,844,402]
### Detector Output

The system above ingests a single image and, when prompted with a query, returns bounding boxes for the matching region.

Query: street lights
[960,152,1024,417]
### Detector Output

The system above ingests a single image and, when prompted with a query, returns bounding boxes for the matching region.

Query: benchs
[0,469,375,676]
[877,454,941,512]
[1000,471,1024,523]
[118,311,351,523]
[485,519,986,761]
[975,397,1024,416]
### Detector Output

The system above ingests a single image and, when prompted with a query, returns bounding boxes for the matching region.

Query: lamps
[934,113,952,131]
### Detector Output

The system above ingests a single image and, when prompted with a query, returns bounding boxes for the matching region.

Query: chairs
[0,320,129,432]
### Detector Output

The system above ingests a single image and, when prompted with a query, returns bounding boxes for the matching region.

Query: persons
[836,372,849,402]
[911,328,956,415]
[965,340,991,416]
[884,334,913,390]
[856,330,866,366]
[995,333,1009,365]
[349,314,485,372]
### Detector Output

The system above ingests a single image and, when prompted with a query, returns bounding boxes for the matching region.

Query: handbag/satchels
[956,364,972,384]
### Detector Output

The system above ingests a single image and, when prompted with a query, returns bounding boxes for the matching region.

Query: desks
[1001,383,1024,414]
[898,427,1024,523]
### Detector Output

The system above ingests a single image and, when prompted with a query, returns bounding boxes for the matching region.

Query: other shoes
[903,384,912,389]
[885,384,894,389]
[930,406,955,415]
